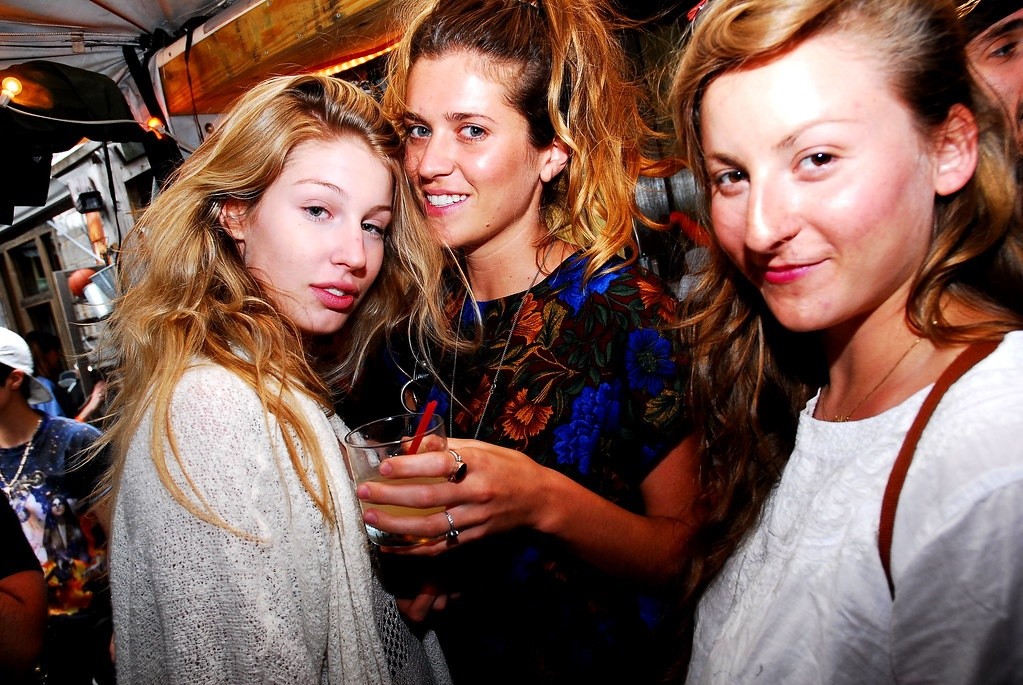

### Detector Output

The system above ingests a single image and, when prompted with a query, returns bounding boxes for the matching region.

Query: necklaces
[0,414,45,499]
[818,294,953,427]
[450,241,554,440]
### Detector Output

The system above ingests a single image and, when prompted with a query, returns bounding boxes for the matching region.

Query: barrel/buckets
[75,320,119,365]
[83,281,114,318]
[72,299,97,321]
[88,262,119,300]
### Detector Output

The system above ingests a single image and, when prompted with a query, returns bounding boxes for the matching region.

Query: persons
[0,0,1023,684]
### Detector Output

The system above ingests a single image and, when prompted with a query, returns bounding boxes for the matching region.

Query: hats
[0,327,54,405]
[953,0,1023,45]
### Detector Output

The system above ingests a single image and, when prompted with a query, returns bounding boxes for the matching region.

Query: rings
[443,510,461,539]
[445,448,467,483]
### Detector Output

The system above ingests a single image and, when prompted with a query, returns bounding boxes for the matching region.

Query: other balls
[70,269,97,298]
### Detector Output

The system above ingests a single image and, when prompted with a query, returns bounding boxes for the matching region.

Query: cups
[345,413,447,547]
